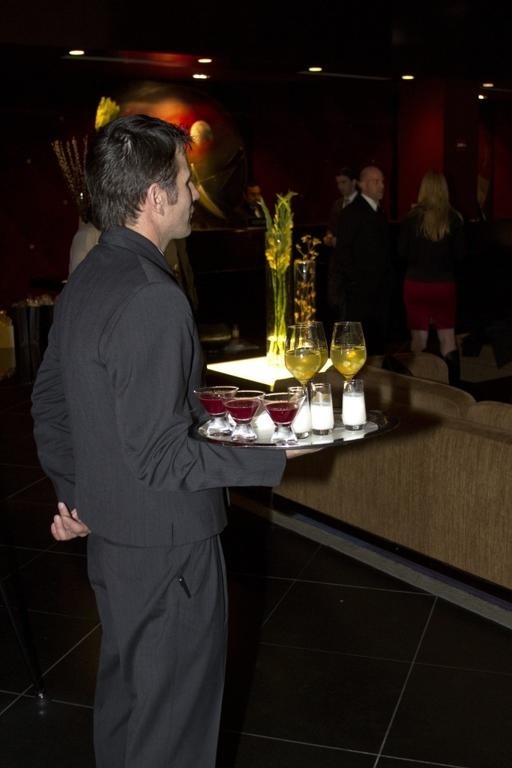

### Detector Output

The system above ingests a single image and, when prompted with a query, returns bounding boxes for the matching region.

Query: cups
[283,321,369,439]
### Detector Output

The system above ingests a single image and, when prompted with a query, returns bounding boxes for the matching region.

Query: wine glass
[192,385,305,445]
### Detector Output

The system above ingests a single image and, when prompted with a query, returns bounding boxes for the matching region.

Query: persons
[317,162,362,314]
[30,116,330,765]
[391,168,462,364]
[69,180,201,313]
[336,164,395,367]
[235,180,267,223]
